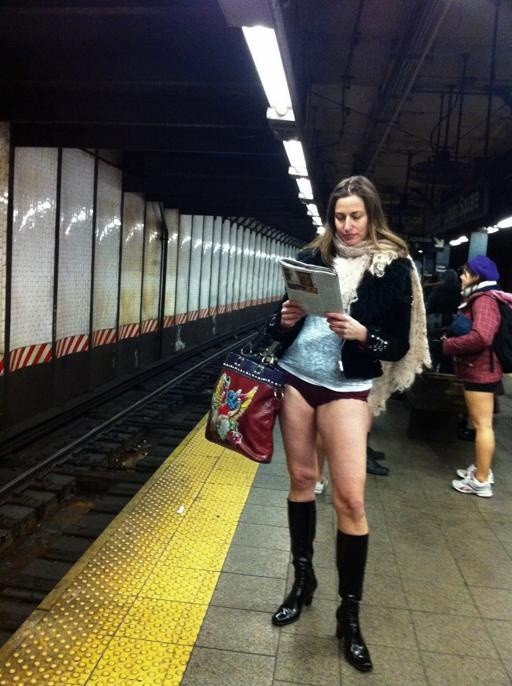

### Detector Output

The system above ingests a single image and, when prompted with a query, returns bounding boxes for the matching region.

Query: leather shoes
[367,455,387,475]
[367,446,386,458]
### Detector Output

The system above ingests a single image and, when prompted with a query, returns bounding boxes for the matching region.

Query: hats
[467,254,499,280]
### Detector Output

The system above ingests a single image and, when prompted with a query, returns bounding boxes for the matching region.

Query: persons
[310,432,329,495]
[365,429,389,476]
[270,174,415,675]
[439,256,507,499]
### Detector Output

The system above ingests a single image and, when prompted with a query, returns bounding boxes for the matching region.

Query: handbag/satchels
[204,351,286,465]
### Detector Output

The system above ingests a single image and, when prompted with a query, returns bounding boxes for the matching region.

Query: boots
[271,500,317,626]
[334,530,373,673]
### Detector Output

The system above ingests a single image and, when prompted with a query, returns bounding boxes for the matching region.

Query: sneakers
[456,463,496,483]
[452,474,493,497]
[315,478,328,494]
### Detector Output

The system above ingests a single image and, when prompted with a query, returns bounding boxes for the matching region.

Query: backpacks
[469,294,512,372]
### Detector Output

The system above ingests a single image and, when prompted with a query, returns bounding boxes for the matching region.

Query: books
[276,256,345,320]
